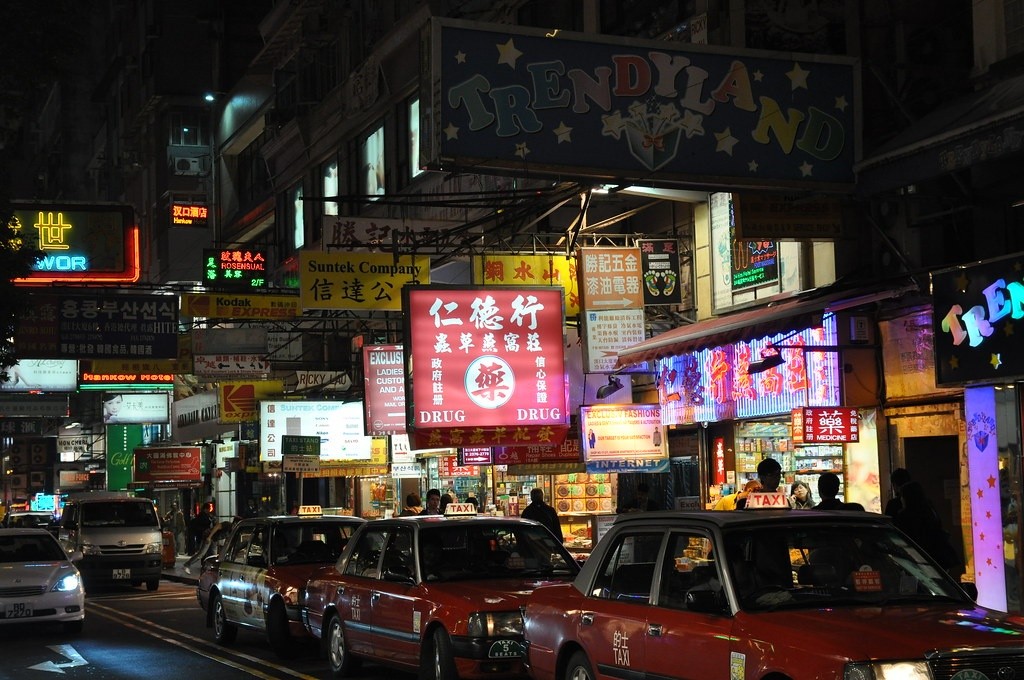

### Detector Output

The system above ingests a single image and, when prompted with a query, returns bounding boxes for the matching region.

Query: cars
[0,527,87,638]
[301,514,583,680]
[521,507,1024,680]
[197,515,388,659]
[6,511,59,534]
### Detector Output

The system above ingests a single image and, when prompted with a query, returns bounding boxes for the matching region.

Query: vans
[58,492,167,591]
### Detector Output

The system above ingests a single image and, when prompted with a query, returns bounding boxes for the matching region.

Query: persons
[400,487,479,517]
[810,472,867,588]
[161,499,263,575]
[713,480,762,511]
[885,468,915,520]
[897,482,962,584]
[521,489,562,564]
[737,458,794,586]
[788,482,815,509]
